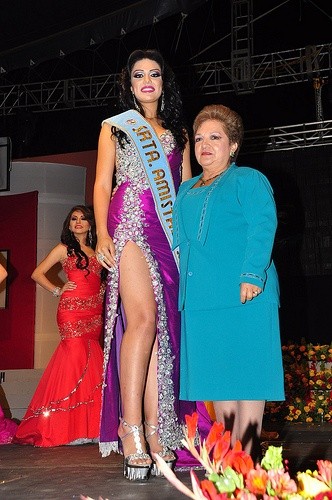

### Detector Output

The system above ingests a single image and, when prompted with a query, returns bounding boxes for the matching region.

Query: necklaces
[199,170,228,188]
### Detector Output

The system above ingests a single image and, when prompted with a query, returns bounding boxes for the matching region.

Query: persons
[93,50,192,481]
[30,205,109,446]
[170,104,286,470]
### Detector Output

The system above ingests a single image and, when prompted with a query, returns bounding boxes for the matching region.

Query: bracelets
[52,286,61,296]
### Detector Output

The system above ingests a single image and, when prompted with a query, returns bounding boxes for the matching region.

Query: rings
[253,291,257,294]
[97,253,105,262]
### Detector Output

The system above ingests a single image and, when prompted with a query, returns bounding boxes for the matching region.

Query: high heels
[117,417,176,482]
